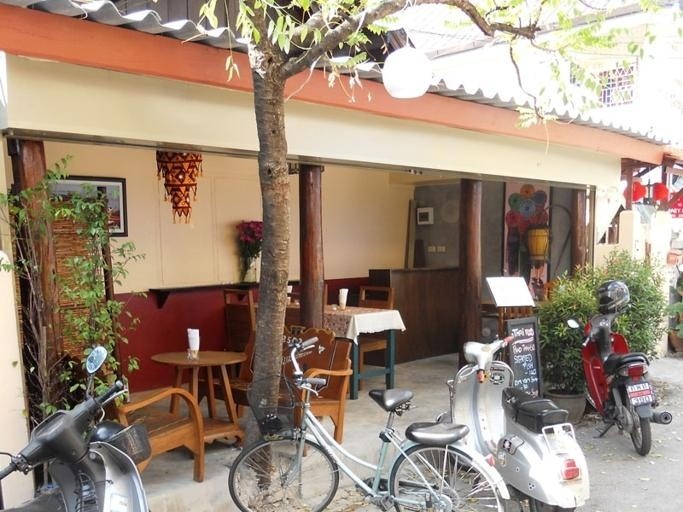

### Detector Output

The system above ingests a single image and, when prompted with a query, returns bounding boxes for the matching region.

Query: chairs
[358,285,395,391]
[223,289,256,378]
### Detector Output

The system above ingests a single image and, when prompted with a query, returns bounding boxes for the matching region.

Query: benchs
[72,355,205,482]
[189,325,354,456]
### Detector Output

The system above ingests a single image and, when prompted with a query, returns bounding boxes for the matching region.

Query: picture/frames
[47,174,128,237]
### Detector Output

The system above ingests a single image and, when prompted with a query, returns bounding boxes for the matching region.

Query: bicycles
[228,337,509,512]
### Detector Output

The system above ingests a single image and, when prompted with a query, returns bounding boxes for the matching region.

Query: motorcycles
[0,347,148,512]
[567,282,672,458]
[434,337,590,512]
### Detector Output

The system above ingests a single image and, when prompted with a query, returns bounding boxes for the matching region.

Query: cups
[186,336,200,360]
[338,295,348,311]
[286,297,292,306]
[331,303,338,311]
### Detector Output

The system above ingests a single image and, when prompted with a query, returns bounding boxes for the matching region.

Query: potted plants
[663,274,683,352]
[533,269,601,425]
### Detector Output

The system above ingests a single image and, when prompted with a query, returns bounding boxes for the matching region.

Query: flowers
[235,221,263,281]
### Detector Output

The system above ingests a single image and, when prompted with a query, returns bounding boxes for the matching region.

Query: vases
[240,255,257,283]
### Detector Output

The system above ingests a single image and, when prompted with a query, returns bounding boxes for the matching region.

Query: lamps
[383,0,432,99]
